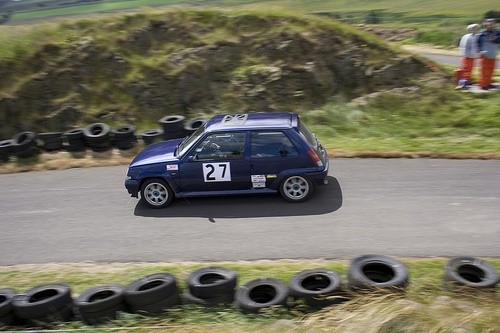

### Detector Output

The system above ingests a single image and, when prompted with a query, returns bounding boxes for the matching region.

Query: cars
[124,112,330,209]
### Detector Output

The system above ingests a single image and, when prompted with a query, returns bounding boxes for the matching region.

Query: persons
[211,134,245,154]
[477,19,500,90]
[459,24,480,85]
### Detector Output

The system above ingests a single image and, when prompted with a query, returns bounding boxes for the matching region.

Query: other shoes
[482,85,495,91]
